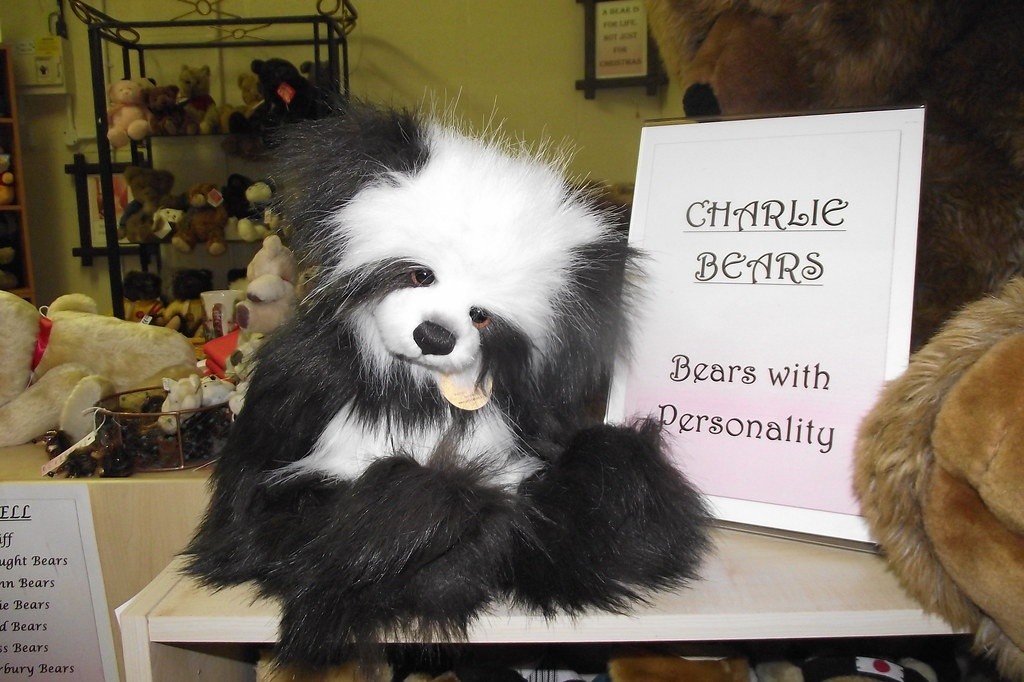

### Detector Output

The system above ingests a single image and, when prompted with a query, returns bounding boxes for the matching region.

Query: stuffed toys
[850,273,1024,682]
[643,0,1024,358]
[0,58,331,451]
[254,637,964,682]
[172,93,719,682]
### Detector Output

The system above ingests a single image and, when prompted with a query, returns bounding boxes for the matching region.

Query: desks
[2,443,236,682]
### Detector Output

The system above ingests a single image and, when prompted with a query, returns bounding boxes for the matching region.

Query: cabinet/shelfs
[63,1,357,327]
[114,512,1024,682]
[1,43,33,302]
